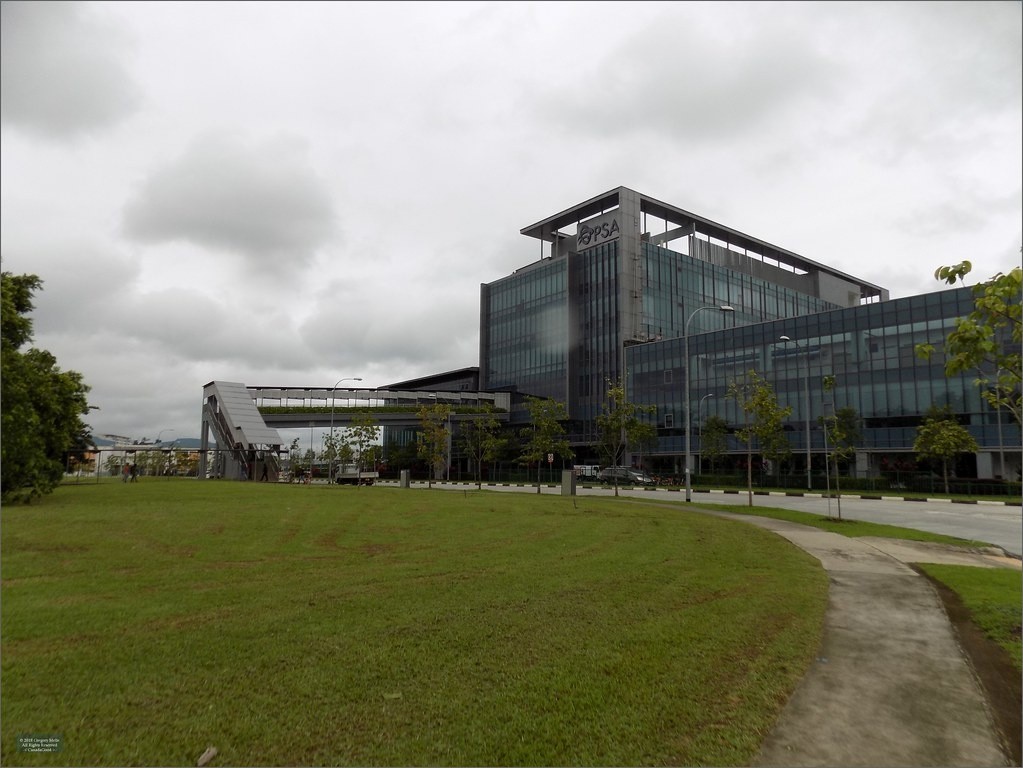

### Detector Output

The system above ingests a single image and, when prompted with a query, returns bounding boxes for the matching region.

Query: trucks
[333,464,379,486]
[574,465,601,482]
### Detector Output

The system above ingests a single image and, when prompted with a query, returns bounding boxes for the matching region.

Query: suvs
[599,466,654,487]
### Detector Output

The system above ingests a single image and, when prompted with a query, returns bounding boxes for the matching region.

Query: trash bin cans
[561,470,576,495]
[400,469,410,488]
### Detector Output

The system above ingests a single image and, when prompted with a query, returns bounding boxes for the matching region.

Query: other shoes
[266,480,268,481]
[261,479,263,481]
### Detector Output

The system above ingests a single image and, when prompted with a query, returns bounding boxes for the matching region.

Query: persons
[297,468,306,483]
[121,463,129,484]
[261,463,269,481]
[130,464,137,482]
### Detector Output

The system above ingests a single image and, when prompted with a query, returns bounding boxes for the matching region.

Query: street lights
[328,377,363,484]
[699,392,713,475]
[155,429,174,476]
[685,306,735,502]
[823,403,833,491]
[780,335,811,491]
[995,366,1005,484]
[428,393,450,482]
